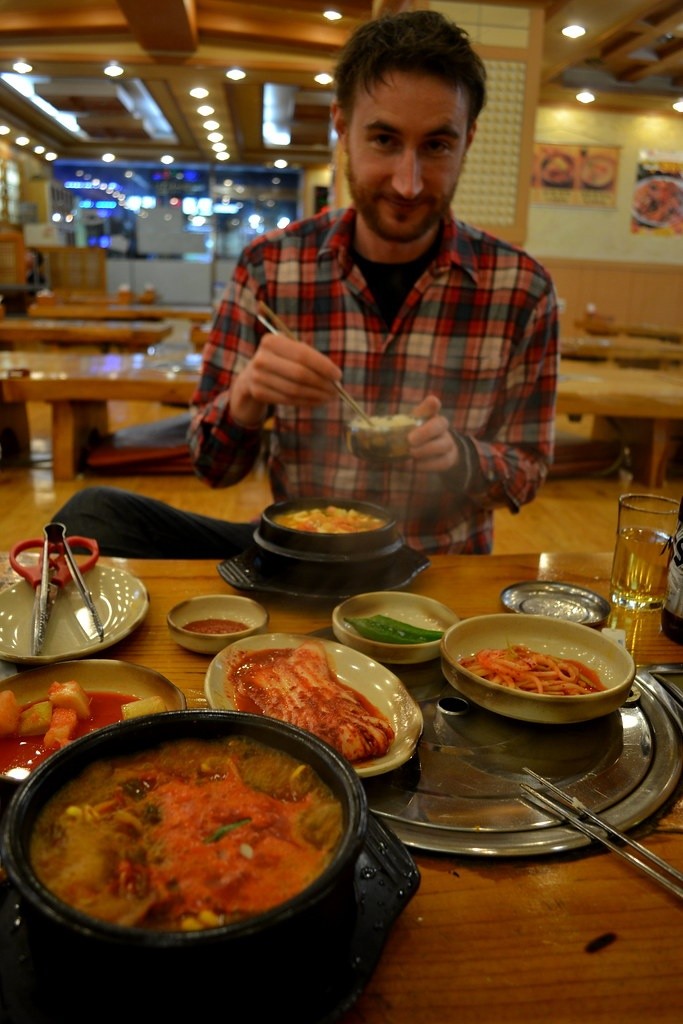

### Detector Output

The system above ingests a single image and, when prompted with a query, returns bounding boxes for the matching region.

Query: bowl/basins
[347,414,424,462]
[0,710,372,983]
[251,495,404,592]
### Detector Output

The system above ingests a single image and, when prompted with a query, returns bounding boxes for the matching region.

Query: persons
[45,9,560,559]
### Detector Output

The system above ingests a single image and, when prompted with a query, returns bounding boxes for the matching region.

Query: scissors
[8,535,99,655]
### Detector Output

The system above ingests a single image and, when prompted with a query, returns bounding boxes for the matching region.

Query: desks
[555,320,683,492]
[1,351,202,481]
[0,289,215,353]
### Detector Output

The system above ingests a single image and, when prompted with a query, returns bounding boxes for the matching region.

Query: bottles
[660,496,683,646]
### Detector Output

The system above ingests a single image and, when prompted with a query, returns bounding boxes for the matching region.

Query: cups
[610,492,681,611]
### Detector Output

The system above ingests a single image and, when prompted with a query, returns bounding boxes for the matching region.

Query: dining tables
[0,550,682,1023]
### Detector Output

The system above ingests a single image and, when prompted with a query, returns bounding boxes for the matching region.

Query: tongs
[29,522,105,656]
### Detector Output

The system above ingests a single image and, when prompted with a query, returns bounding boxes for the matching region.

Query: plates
[500,580,611,627]
[579,160,613,189]
[0,814,420,1024]
[165,593,269,656]
[204,634,422,776]
[438,611,637,722]
[0,564,150,668]
[632,176,683,226]
[540,153,575,186]
[0,658,187,792]
[331,592,462,665]
[216,543,432,602]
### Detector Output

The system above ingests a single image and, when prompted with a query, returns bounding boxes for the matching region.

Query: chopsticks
[256,301,375,427]
[520,767,683,902]
